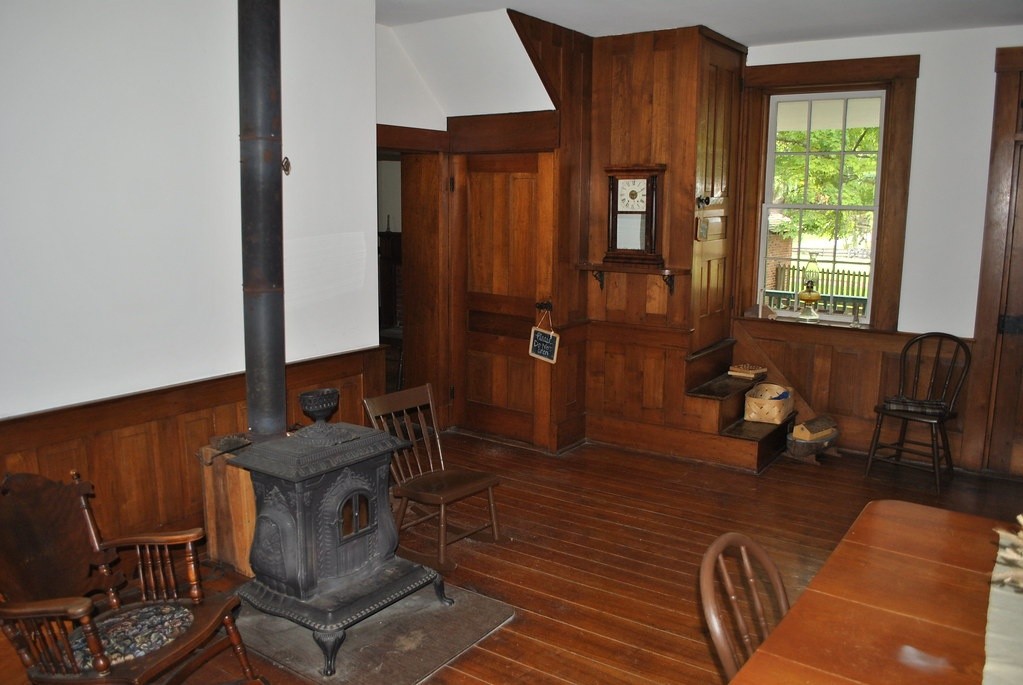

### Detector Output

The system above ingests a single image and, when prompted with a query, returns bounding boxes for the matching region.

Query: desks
[729,501,1023,685]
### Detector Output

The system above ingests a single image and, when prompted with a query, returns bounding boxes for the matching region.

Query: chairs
[361,381,501,565]
[700,532,790,682]
[865,332,971,498]
[0,469,272,685]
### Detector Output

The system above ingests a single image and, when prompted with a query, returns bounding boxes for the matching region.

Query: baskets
[744,380,796,424]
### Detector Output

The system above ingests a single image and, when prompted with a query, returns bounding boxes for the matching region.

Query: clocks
[602,164,666,269]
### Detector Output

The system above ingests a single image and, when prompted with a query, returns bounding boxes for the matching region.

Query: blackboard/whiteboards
[529,326,560,364]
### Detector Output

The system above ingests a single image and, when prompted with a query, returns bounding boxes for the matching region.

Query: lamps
[798,252,822,317]
